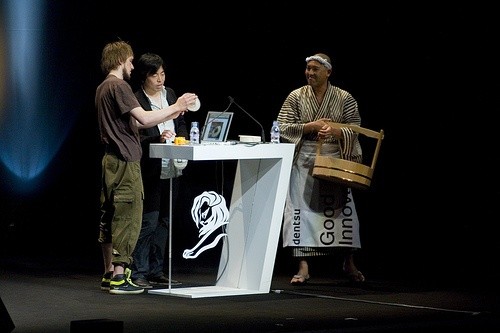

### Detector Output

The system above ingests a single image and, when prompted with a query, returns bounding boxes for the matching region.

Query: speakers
[69,318,124,333]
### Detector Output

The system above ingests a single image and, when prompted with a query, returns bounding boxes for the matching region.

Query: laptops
[199,112,233,144]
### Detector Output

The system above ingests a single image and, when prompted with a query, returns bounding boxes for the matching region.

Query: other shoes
[342,268,365,282]
[291,273,310,285]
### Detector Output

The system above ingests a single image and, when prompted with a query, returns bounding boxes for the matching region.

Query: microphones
[198,96,265,143]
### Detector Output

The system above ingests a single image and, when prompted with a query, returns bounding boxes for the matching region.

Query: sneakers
[101,271,114,290]
[109,267,144,295]
[149,274,183,285]
[132,276,153,288]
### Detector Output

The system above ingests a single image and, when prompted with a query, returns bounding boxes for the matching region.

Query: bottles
[270,121,281,143]
[190,122,199,144]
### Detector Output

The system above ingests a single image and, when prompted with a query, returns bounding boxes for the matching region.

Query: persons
[131,52,189,288]
[277,53,367,284]
[95,41,198,296]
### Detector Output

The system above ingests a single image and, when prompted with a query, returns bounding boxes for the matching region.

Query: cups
[174,137,185,145]
[187,97,201,112]
